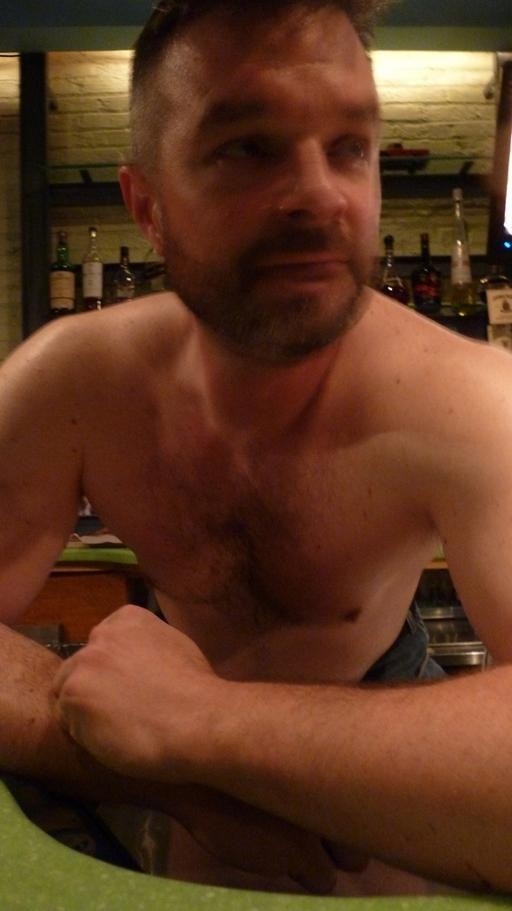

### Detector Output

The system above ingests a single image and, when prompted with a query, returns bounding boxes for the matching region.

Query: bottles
[376,185,510,338]
[43,228,135,319]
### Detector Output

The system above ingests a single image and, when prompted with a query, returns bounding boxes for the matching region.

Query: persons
[1,2,511,895]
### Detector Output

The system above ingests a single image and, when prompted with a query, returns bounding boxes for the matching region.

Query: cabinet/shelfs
[18,174,509,340]
